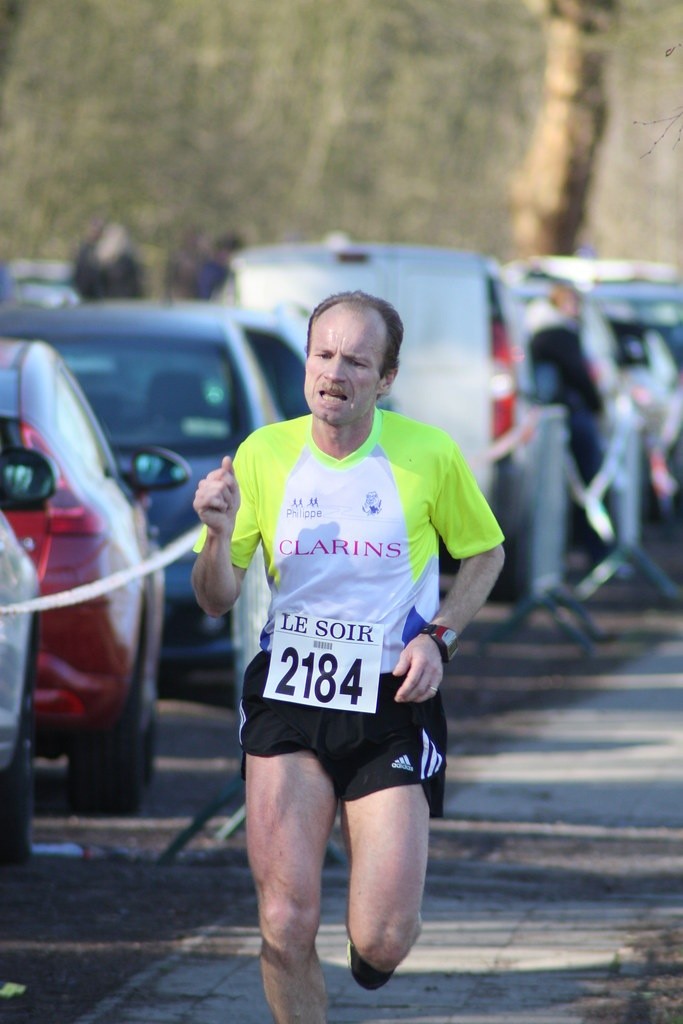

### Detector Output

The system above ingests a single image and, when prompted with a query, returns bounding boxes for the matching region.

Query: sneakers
[347,939,395,991]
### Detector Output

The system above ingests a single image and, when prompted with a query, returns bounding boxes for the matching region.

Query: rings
[429,686,438,692]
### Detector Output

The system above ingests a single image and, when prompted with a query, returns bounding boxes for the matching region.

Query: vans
[220,236,553,597]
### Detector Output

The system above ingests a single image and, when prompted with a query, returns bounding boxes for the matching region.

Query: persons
[69,216,243,304]
[190,291,505,1024]
[525,283,635,580]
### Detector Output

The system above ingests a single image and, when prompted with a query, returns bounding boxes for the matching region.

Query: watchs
[419,623,459,663]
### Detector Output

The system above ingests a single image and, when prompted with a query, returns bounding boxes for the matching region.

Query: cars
[503,254,683,555]
[0,444,55,859]
[0,337,193,814]
[0,298,310,695]
